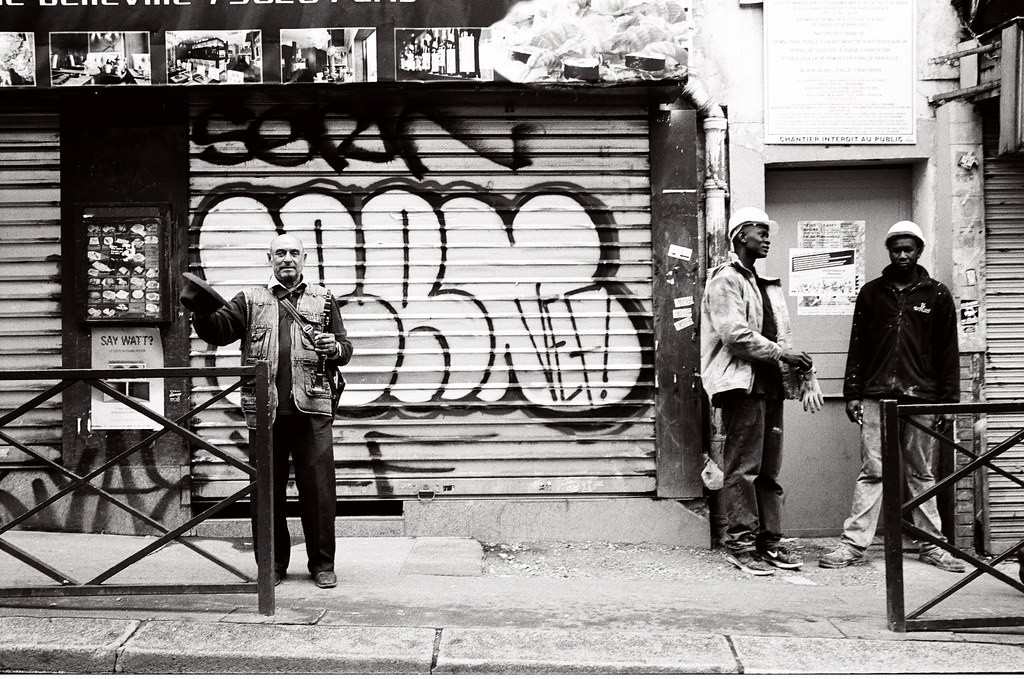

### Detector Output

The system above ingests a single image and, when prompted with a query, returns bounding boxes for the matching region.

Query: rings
[326,344,327,348]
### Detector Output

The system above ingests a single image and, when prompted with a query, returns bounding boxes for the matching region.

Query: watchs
[327,341,341,357]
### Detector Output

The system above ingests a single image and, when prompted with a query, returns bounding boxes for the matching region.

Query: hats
[180,272,234,318]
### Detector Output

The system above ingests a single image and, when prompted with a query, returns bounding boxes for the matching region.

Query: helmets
[727,207,779,240]
[886,220,925,255]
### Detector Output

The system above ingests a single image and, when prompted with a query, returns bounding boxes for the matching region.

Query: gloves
[799,365,824,414]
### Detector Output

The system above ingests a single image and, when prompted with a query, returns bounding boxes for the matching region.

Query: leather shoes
[274,570,287,586]
[311,570,338,588]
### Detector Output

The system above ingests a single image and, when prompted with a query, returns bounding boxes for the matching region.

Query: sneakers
[726,551,775,575]
[759,547,803,569]
[920,548,966,572]
[819,543,866,568]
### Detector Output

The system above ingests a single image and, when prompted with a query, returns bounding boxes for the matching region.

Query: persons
[116,58,136,84]
[192,234,353,587]
[819,220,966,571]
[701,207,817,575]
[805,296,819,307]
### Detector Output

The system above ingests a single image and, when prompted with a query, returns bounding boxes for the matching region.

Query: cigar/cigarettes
[857,416,862,425]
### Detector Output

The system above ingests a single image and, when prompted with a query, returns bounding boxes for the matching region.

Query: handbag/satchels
[326,364,346,425]
